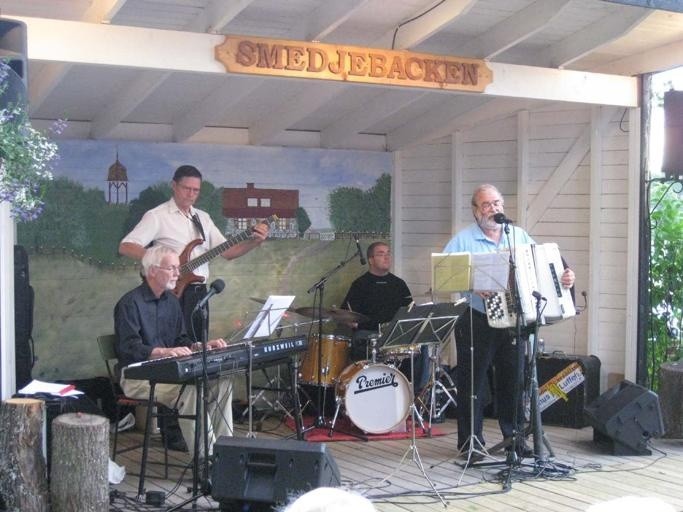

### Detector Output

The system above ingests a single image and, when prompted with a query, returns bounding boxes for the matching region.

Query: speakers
[212,436,341,512]
[582,378,665,455]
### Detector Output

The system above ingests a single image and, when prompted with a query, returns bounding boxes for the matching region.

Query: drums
[380,321,421,363]
[351,330,381,358]
[335,359,414,435]
[297,332,352,388]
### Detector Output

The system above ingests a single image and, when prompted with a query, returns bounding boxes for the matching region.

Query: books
[430,251,509,292]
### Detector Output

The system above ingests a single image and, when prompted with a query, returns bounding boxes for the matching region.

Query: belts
[186,285,206,291]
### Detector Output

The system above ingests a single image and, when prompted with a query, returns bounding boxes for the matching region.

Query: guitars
[170,214,279,298]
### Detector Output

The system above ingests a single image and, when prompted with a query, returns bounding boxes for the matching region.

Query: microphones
[353,233,367,266]
[194,278,226,312]
[580,290,588,312]
[494,213,514,224]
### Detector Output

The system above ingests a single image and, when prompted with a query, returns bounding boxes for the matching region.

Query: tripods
[462,225,570,492]
[462,335,557,466]
[238,254,369,443]
[429,292,505,488]
[362,349,450,509]
[417,343,458,424]
[164,313,212,512]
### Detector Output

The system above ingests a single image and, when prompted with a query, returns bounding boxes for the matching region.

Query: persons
[442,185,576,465]
[114,247,234,479]
[340,242,416,332]
[119,165,269,451]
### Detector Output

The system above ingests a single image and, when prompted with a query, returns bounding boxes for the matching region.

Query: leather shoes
[505,439,535,458]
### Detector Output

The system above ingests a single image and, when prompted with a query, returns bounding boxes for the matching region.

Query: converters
[146,491,164,506]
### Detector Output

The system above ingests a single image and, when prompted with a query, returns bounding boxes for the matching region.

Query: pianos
[124,335,309,383]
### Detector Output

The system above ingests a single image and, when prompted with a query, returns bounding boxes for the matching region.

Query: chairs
[96,332,171,480]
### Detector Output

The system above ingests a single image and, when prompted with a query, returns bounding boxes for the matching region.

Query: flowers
[1,52,70,226]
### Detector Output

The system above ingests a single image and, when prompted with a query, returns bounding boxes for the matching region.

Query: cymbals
[297,306,370,328]
[250,297,297,314]
